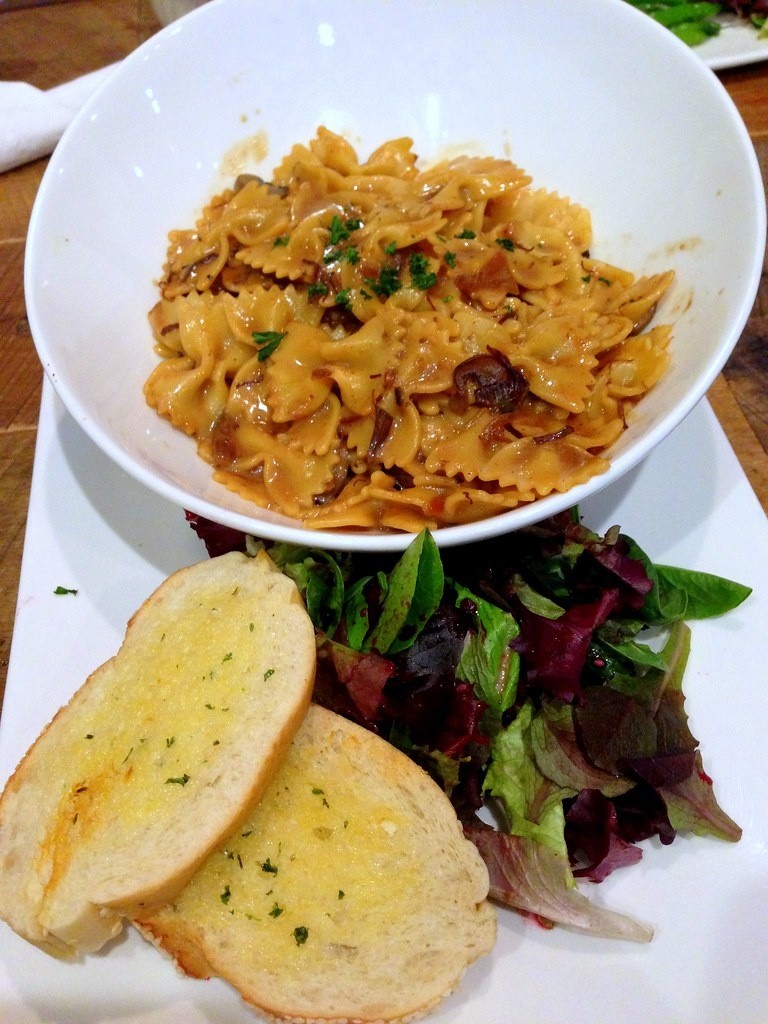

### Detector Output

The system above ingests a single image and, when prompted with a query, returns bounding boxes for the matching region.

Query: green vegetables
[265,508,751,943]
[624,0,723,44]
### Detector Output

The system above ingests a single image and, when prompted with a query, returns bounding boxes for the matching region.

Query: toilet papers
[0,59,124,174]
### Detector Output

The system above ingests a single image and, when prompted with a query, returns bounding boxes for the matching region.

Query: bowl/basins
[23,1,768,553]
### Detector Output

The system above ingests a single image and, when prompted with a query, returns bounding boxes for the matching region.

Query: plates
[641,6,768,71]
[0,375,768,1024]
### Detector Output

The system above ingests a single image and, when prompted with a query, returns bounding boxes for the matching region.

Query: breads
[128,705,495,1024]
[0,546,318,963]
